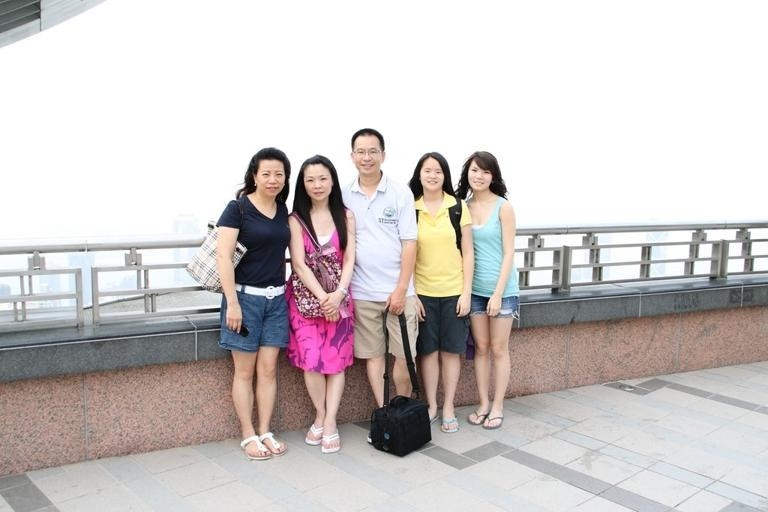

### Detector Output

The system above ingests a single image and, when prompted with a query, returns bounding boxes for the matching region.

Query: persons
[407,152,475,434]
[217,149,290,461]
[344,129,419,441]
[454,152,521,430]
[285,154,356,454]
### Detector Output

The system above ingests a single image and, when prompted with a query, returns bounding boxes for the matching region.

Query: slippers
[241,436,271,460]
[468,410,489,425]
[441,418,459,433]
[430,415,439,425]
[260,431,287,457]
[483,414,504,430]
[305,424,323,445]
[321,429,341,453]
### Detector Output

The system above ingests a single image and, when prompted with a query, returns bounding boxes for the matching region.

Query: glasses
[353,150,381,158]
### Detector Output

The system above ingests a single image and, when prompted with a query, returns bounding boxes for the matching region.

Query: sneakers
[367,431,372,444]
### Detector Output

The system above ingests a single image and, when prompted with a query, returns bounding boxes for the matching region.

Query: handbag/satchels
[186,200,247,294]
[288,212,351,319]
[370,305,432,457]
